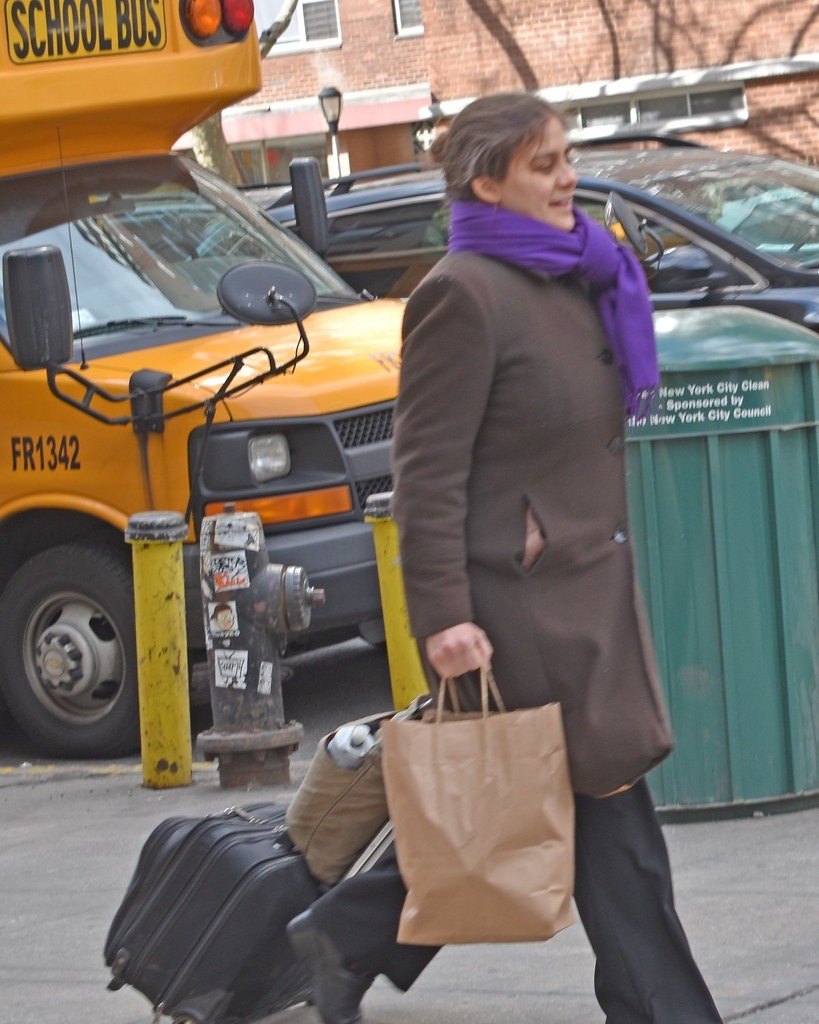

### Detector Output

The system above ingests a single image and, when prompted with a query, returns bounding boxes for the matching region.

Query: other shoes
[285,909,380,1024]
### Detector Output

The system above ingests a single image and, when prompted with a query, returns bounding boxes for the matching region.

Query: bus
[0,0,408,756]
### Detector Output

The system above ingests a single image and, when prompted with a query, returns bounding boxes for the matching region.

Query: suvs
[89,132,819,334]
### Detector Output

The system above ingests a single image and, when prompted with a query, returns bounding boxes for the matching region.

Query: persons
[288,94,730,1024]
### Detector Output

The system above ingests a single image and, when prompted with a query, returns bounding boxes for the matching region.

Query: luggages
[103,698,433,1024]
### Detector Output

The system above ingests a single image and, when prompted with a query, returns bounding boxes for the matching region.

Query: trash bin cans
[620,304,819,821]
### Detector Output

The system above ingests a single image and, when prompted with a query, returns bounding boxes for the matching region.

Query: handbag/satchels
[382,666,576,946]
[286,711,398,884]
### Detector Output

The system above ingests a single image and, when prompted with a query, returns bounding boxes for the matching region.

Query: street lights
[318,85,342,176]
